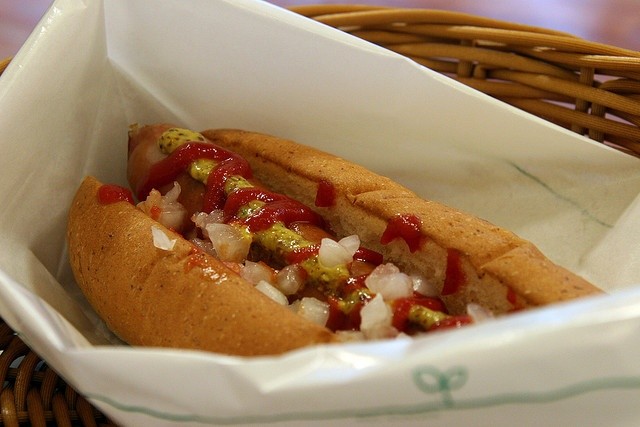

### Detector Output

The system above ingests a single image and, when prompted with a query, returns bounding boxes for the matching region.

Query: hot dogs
[66,123,608,357]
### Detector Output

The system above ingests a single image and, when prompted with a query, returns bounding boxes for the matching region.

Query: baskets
[1,1,640,427]
